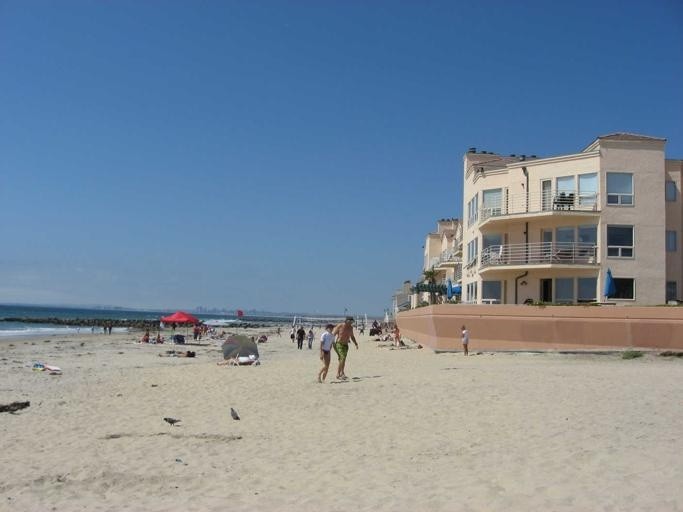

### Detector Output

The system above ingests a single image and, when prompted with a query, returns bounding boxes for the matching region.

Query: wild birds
[164,418,181,426]
[231,408,240,420]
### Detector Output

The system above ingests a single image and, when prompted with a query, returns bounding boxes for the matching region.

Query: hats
[325,324,336,329]
[345,317,354,323]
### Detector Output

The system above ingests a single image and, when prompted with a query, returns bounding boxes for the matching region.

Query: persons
[77,321,81,332]
[458,325,469,357]
[391,325,401,350]
[316,323,340,384]
[200,324,216,336]
[142,324,150,344]
[276,326,281,338]
[91,323,94,334]
[359,324,364,336]
[158,349,195,357]
[371,320,381,329]
[102,321,112,336]
[332,315,359,382]
[191,322,198,340]
[289,325,314,350]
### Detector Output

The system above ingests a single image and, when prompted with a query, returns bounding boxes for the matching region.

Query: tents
[158,313,200,343]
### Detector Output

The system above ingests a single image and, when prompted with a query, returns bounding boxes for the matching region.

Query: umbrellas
[604,267,617,303]
[221,334,259,368]
[445,278,453,303]
